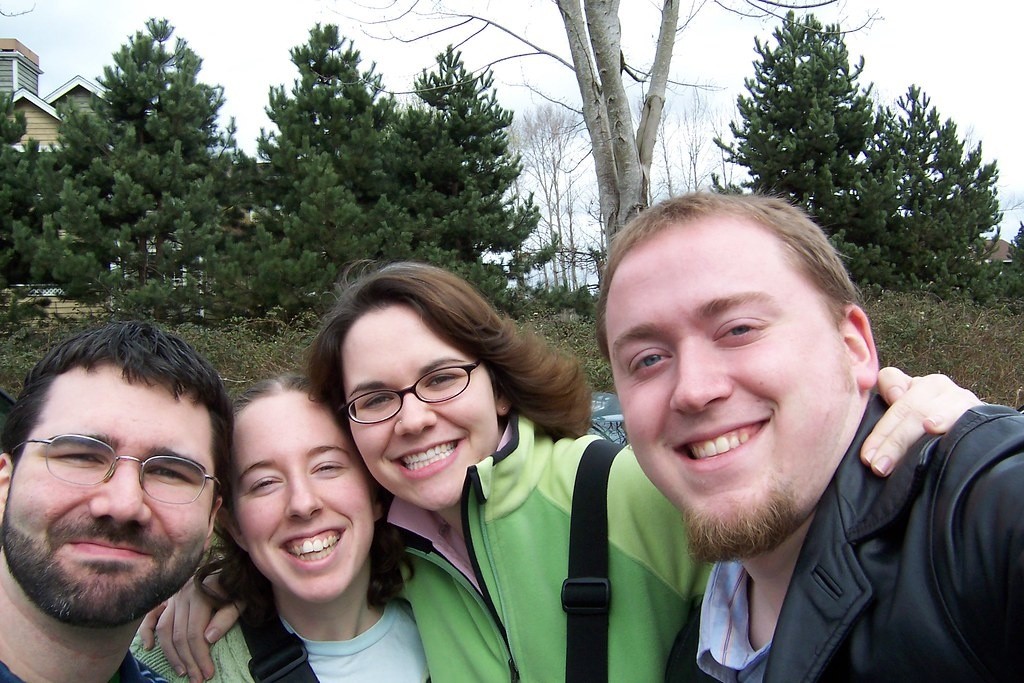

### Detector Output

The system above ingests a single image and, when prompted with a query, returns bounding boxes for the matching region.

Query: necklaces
[353,594,367,638]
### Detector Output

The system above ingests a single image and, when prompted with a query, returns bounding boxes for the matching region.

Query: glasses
[11,434,222,505]
[339,357,484,425]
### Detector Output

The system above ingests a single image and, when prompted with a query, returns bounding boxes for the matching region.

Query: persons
[0,317,234,683]
[596,191,1024,683]
[133,262,985,683]
